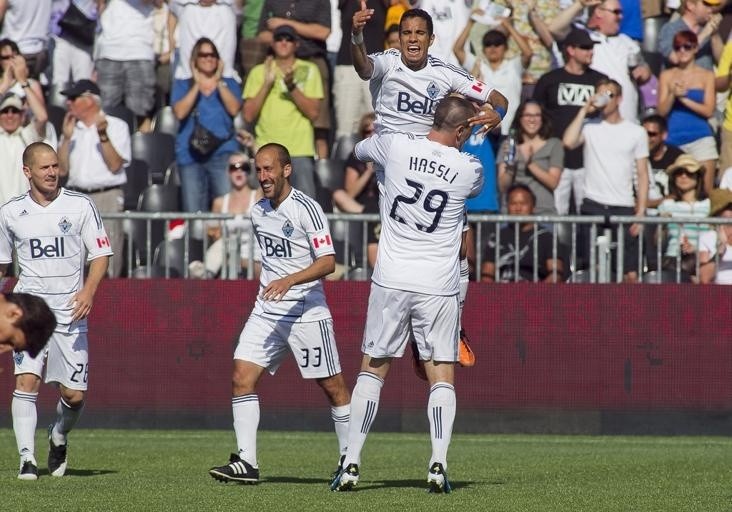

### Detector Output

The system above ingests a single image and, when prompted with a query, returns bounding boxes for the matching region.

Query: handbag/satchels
[190,122,221,162]
[57,5,97,40]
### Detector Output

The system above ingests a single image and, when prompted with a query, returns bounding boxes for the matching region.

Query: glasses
[230,162,250,173]
[675,44,692,52]
[198,52,216,59]
[648,131,661,137]
[601,8,623,15]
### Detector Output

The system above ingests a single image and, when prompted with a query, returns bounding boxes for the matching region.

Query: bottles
[593,90,614,109]
[507,126,516,166]
[680,222,691,253]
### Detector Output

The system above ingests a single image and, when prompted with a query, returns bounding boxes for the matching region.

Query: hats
[60,80,101,97]
[564,28,601,46]
[666,154,703,173]
[0,93,24,111]
[273,25,297,40]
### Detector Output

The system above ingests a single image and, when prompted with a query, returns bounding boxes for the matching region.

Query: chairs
[117,123,731,285]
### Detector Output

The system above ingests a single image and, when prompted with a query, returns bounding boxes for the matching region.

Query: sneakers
[426,463,452,492]
[329,455,359,492]
[18,460,38,480]
[46,424,69,477]
[212,457,260,484]
[458,338,475,368]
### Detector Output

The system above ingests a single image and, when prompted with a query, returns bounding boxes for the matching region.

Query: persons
[0,141,115,480]
[419,0,732,284]
[349,2,508,382]
[0,293,57,359]
[209,142,352,485]
[1,1,421,279]
[331,94,485,494]
